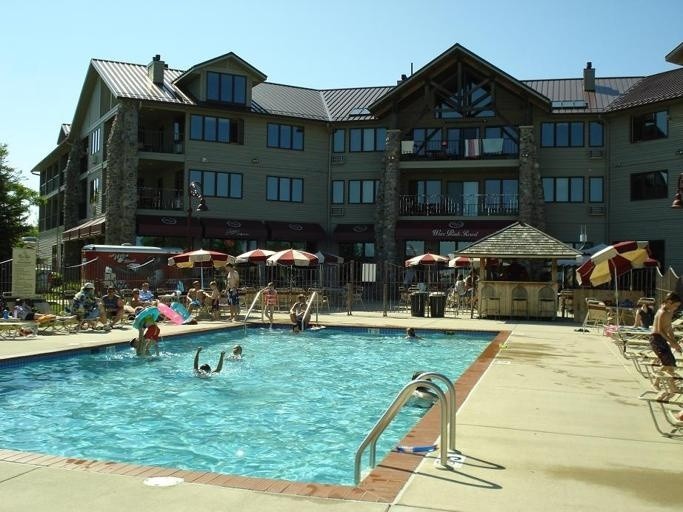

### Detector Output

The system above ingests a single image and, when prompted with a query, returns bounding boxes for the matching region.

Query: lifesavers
[158,303,184,324]
[133,306,160,329]
[170,302,191,320]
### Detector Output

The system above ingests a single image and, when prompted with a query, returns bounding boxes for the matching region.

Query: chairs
[400,285,478,308]
[257,287,280,311]
[308,288,330,314]
[343,284,365,309]
[584,295,683,439]
[0,288,248,341]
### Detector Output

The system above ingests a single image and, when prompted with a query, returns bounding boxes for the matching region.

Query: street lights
[671,173,683,208]
[187,181,208,253]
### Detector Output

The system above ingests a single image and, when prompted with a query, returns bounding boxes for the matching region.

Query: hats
[81,282,95,290]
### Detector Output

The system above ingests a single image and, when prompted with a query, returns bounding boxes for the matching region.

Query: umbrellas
[266,248,319,291]
[315,251,348,288]
[404,251,451,291]
[576,240,650,326]
[234,248,277,286]
[444,255,500,317]
[168,248,236,289]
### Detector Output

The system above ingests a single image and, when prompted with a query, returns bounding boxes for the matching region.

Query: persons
[648,292,682,390]
[454,269,478,305]
[632,303,652,329]
[405,328,419,339]
[399,267,417,288]
[0,262,314,373]
[404,372,437,408]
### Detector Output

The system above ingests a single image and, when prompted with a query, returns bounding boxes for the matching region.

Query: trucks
[78,243,194,293]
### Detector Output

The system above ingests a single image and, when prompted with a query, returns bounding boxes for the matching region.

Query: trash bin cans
[410,292,446,317]
